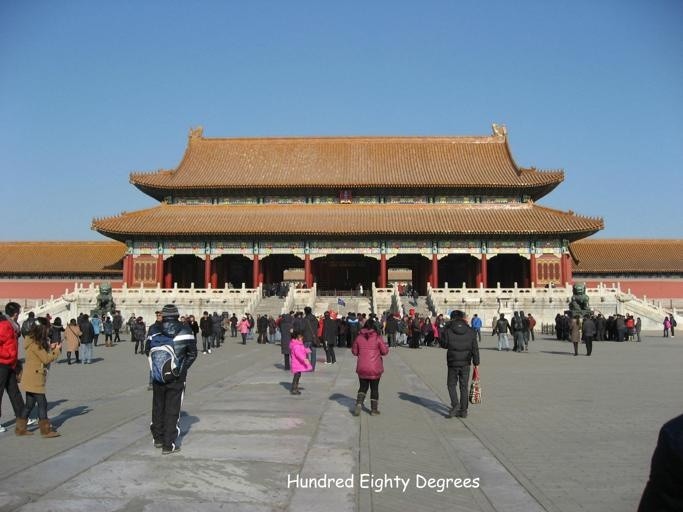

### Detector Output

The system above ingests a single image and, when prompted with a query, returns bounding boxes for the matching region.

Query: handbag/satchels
[312,334,320,347]
[469,382,482,404]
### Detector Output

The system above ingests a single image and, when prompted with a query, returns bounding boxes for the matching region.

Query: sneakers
[162,445,180,454]
[208,349,212,354]
[0,425,6,432]
[203,351,206,354]
[450,402,461,417]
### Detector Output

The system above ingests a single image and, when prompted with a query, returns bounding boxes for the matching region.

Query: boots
[38,419,60,438]
[292,382,301,394]
[16,417,34,435]
[371,399,380,416]
[354,392,366,416]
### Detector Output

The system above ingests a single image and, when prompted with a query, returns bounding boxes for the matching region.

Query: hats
[162,304,180,319]
[155,310,162,314]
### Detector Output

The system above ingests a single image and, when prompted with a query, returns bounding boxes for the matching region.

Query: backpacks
[148,335,187,384]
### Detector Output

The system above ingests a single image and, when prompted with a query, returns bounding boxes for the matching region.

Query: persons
[15,319,62,439]
[351,317,389,417]
[412,289,418,306]
[669,315,677,336]
[582,314,595,356]
[355,279,365,297]
[143,310,162,391]
[438,309,480,419]
[568,314,580,357]
[23,305,470,367]
[288,331,313,395]
[492,314,510,350]
[0,302,36,432]
[635,412,682,511]
[527,313,535,339]
[398,282,413,299]
[262,281,307,299]
[554,312,641,343]
[510,312,523,351]
[470,313,482,342]
[519,310,529,350]
[148,304,198,456]
[491,316,496,335]
[662,317,670,337]
